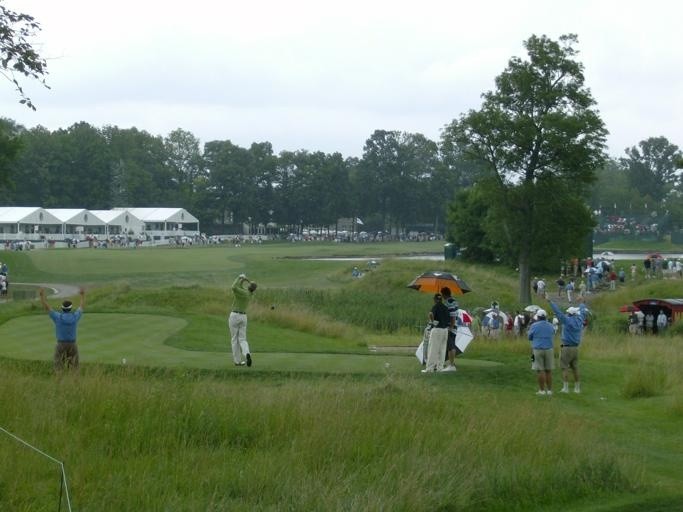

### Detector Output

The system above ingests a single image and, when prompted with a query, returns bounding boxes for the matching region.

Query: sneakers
[536,389,580,395]
[421,365,456,373]
[247,354,251,366]
[235,362,245,366]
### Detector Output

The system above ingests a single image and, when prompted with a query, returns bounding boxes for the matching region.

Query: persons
[420,257,683,394]
[0,262,7,295]
[38,285,85,366]
[0,231,445,251]
[227,274,256,366]
[594,218,658,235]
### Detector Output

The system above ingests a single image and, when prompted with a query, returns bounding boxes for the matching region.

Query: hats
[566,307,576,314]
[537,310,547,317]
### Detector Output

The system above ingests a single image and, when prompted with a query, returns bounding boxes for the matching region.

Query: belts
[563,344,571,347]
[232,311,247,314]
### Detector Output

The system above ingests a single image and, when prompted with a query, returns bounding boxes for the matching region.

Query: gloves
[239,274,246,280]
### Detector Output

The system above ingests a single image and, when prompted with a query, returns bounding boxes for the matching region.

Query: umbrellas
[405,270,473,304]
